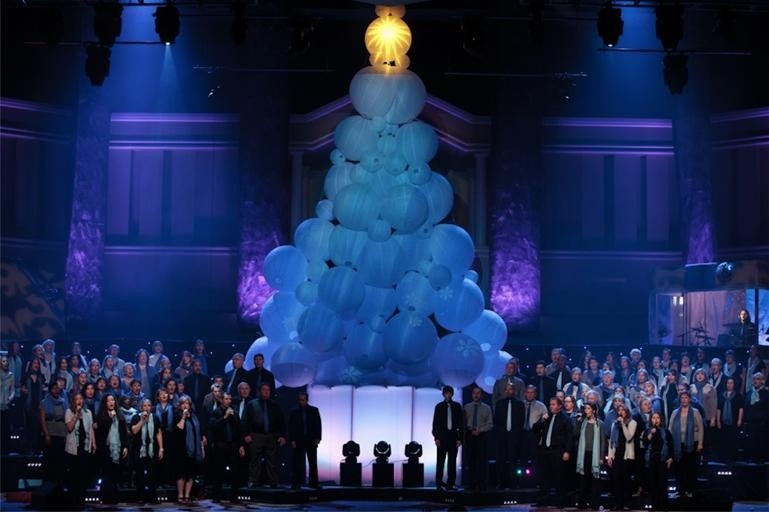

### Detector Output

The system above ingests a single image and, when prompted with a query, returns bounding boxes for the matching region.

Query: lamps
[152,4,180,46]
[654,4,683,49]
[662,49,688,96]
[90,1,125,50]
[598,9,624,47]
[84,44,112,87]
[363,2,412,66]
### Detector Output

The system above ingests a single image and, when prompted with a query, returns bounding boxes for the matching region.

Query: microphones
[227,405,235,416]
[183,407,190,419]
[143,410,148,419]
[579,411,588,424]
[615,411,624,426]
[110,407,116,423]
[651,422,657,434]
[76,403,82,415]
[541,409,552,424]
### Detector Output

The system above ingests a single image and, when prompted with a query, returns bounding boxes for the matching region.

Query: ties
[526,403,531,430]
[557,371,562,390]
[540,377,544,402]
[546,415,556,447]
[263,401,269,432]
[239,398,245,420]
[447,403,453,431]
[473,404,478,428]
[506,400,512,431]
[227,368,239,393]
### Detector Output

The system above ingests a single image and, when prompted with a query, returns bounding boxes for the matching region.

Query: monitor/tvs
[683,263,717,293]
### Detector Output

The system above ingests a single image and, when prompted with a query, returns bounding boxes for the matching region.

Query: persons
[730,309,754,345]
